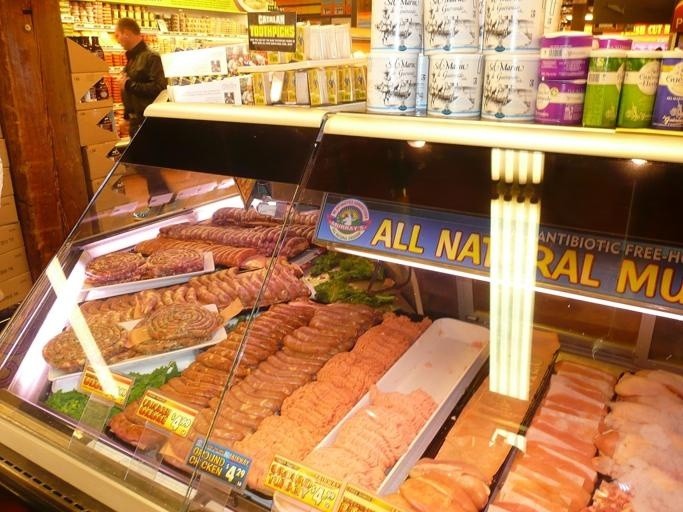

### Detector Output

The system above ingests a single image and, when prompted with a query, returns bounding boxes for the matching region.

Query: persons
[111,17,169,194]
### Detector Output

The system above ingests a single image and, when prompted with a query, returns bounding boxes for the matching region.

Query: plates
[46,304,226,383]
[80,251,215,293]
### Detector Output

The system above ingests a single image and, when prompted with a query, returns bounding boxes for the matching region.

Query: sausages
[80,256,312,322]
[111,298,384,500]
[132,203,322,269]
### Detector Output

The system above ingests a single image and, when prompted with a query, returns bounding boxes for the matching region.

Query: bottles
[65,34,108,103]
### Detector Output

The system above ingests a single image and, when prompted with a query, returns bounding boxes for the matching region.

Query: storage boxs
[67,40,136,231]
